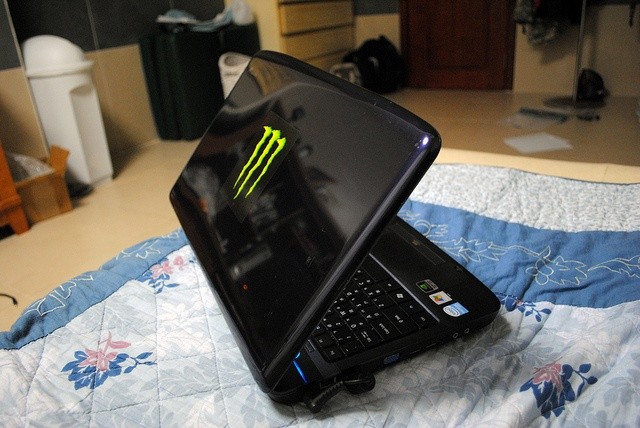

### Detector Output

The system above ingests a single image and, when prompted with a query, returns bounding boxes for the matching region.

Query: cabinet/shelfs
[225,1,354,73]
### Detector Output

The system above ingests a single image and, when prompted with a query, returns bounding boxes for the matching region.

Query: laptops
[169,49,501,412]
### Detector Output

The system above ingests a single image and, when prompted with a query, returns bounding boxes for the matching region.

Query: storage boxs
[13,146,73,225]
